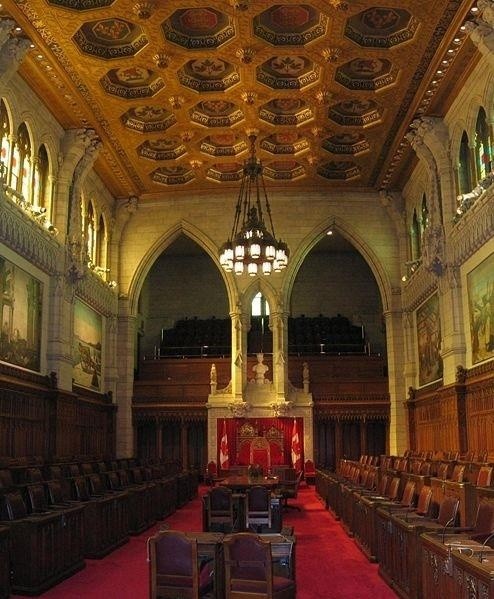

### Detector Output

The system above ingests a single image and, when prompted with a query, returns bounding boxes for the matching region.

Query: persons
[1,321,8,336]
[251,352,269,379]
[11,328,21,341]
[302,362,311,383]
[210,364,217,382]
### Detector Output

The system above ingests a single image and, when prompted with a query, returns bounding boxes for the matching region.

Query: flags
[291,420,301,468]
[219,420,228,470]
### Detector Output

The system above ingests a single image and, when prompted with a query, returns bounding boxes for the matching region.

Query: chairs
[248,437,271,471]
[146,471,305,599]
[315,449,494,599]
[204,461,217,486]
[303,459,318,485]
[0,456,199,599]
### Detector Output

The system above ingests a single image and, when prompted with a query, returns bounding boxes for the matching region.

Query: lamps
[217,135,289,275]
